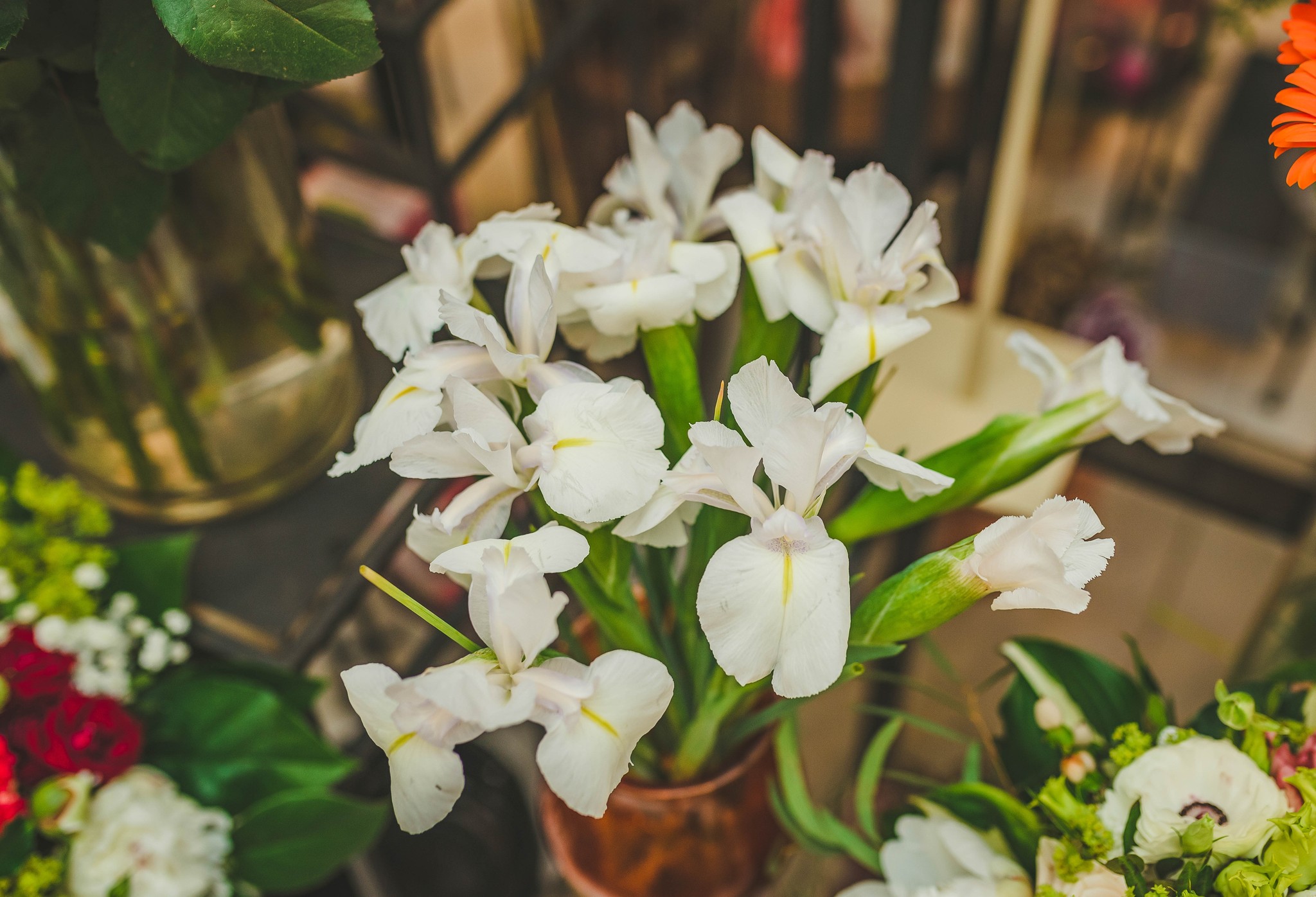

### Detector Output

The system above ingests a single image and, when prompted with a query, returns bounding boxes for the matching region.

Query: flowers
[316,88,1224,842]
[0,461,393,897]
[754,631,1316,897]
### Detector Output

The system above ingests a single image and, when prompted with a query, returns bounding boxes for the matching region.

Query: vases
[533,592,779,897]
